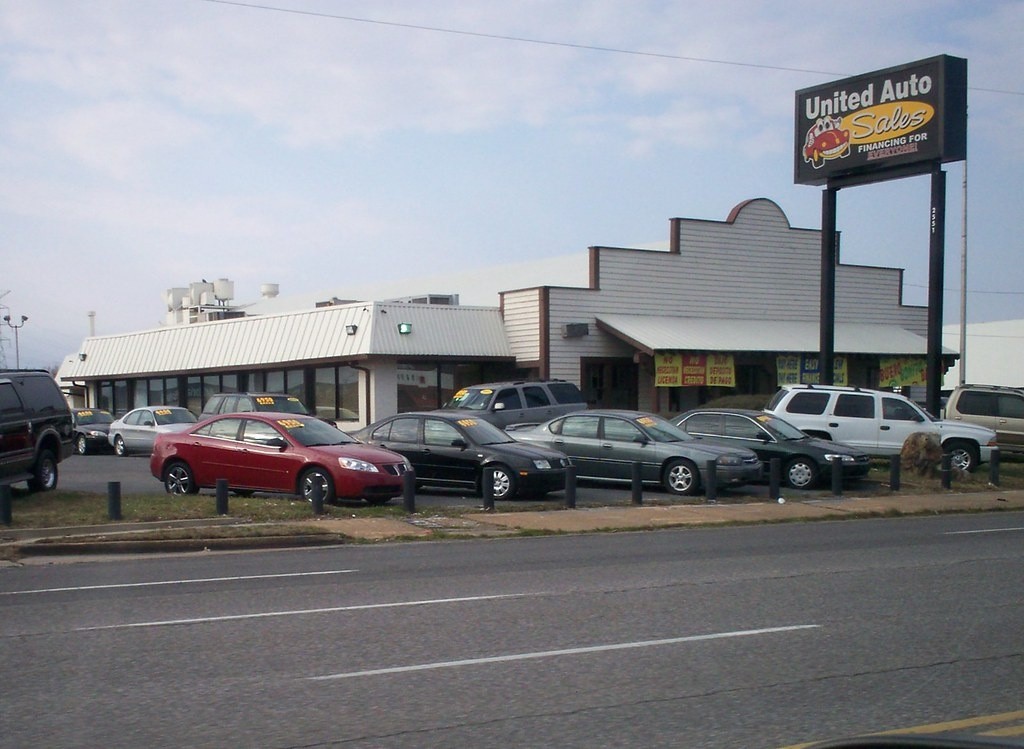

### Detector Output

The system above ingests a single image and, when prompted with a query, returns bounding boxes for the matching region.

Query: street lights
[3,315,28,369]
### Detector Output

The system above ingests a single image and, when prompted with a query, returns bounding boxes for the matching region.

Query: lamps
[79,353,87,361]
[346,325,357,336]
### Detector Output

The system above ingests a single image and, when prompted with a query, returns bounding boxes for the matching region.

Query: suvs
[0,369,75,492]
[939,385,1024,451]
[197,392,338,441]
[417,379,589,429]
[763,385,999,474]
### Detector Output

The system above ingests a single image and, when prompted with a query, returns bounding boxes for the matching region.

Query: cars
[69,408,118,455]
[107,406,198,457]
[150,411,414,505]
[342,410,573,500]
[666,407,872,491]
[503,409,765,497]
